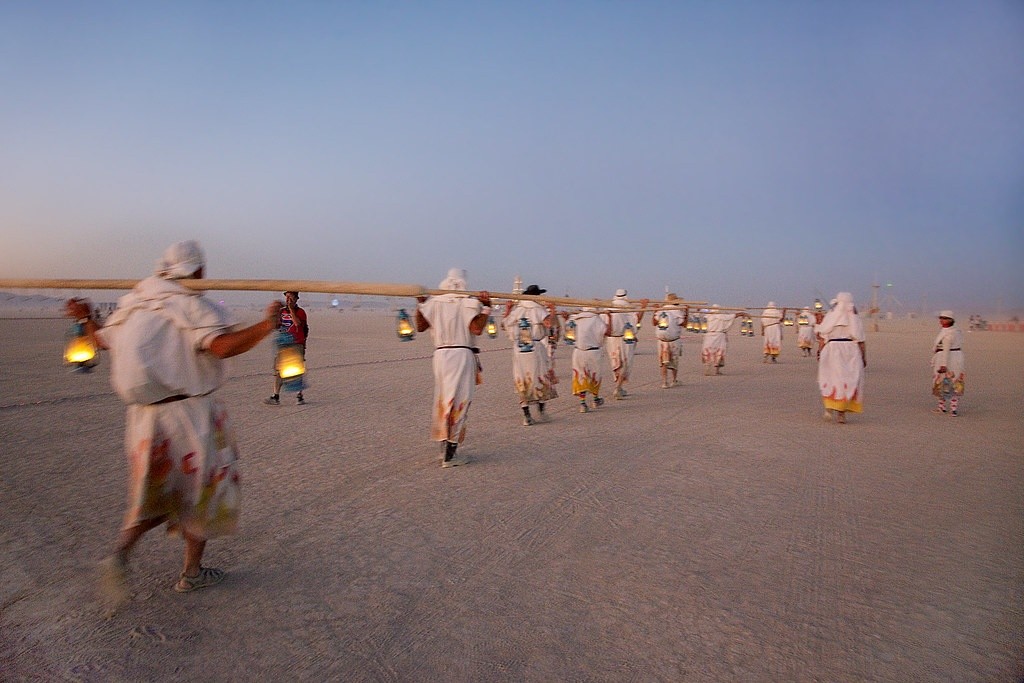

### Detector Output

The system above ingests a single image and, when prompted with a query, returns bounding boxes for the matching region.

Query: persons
[264,290,310,405]
[562,302,612,412]
[501,285,561,425]
[818,291,867,426]
[653,293,689,388]
[762,301,781,364]
[932,310,965,416]
[416,268,492,468]
[66,241,286,593]
[798,306,817,356]
[601,289,649,400]
[702,304,751,376]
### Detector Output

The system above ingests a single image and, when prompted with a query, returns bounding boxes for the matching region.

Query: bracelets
[75,315,91,324]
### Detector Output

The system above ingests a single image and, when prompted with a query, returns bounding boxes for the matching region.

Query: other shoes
[769,360,777,364]
[837,414,847,423]
[594,396,604,409]
[931,407,946,414]
[613,385,628,400]
[763,357,767,364]
[663,382,669,389]
[704,367,724,376]
[263,394,280,407]
[296,395,303,404]
[825,411,835,422]
[524,416,534,426]
[950,410,957,416]
[441,455,470,467]
[670,379,683,386]
[580,403,588,413]
[538,412,548,422]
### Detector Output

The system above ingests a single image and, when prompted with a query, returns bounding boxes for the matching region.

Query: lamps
[563,320,578,346]
[740,317,756,337]
[621,321,637,345]
[62,312,102,377]
[636,322,641,330]
[393,306,417,344]
[685,313,708,334]
[814,298,824,311]
[658,312,669,331]
[484,316,498,339]
[516,317,536,353]
[799,315,809,325]
[784,316,794,328]
[272,324,312,392]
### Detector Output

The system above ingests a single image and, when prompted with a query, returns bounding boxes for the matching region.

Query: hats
[523,284,546,296]
[938,309,954,320]
[663,293,683,304]
[612,290,627,301]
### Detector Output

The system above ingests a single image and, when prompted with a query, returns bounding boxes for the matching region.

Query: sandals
[174,567,225,592]
